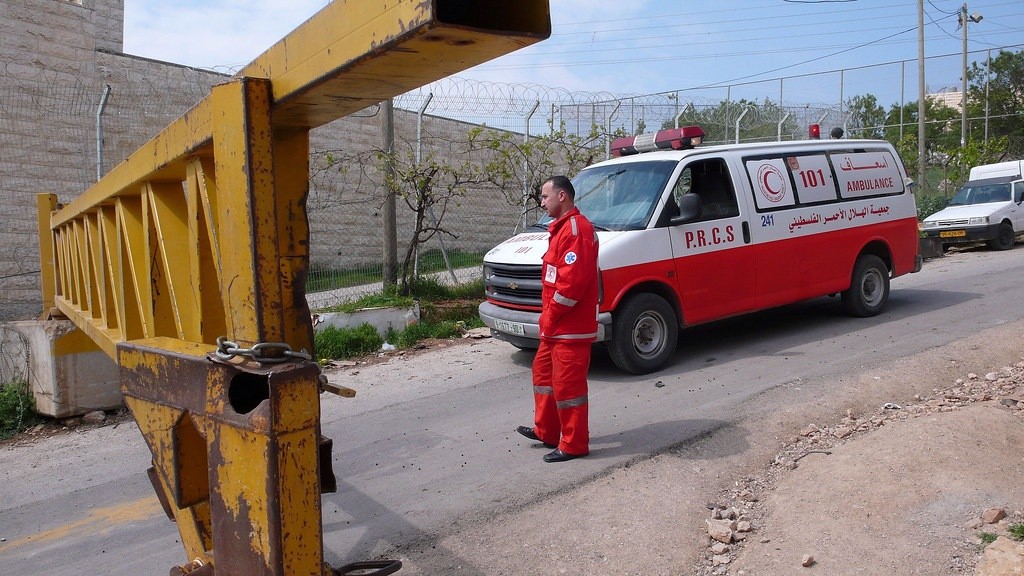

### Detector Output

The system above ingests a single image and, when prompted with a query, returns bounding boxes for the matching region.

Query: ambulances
[479,125,923,375]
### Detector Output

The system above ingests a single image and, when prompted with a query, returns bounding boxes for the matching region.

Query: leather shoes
[517,426,557,448]
[543,447,576,463]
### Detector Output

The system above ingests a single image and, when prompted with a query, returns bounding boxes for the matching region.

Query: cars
[921,175,1024,250]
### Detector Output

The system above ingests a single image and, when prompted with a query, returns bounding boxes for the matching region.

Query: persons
[516,174,603,464]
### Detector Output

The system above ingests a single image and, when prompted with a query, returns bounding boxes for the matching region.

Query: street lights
[843,111,854,139]
[522,99,541,233]
[777,112,791,141]
[415,93,434,281]
[605,101,621,212]
[675,103,688,128]
[95,83,112,182]
[734,108,748,144]
